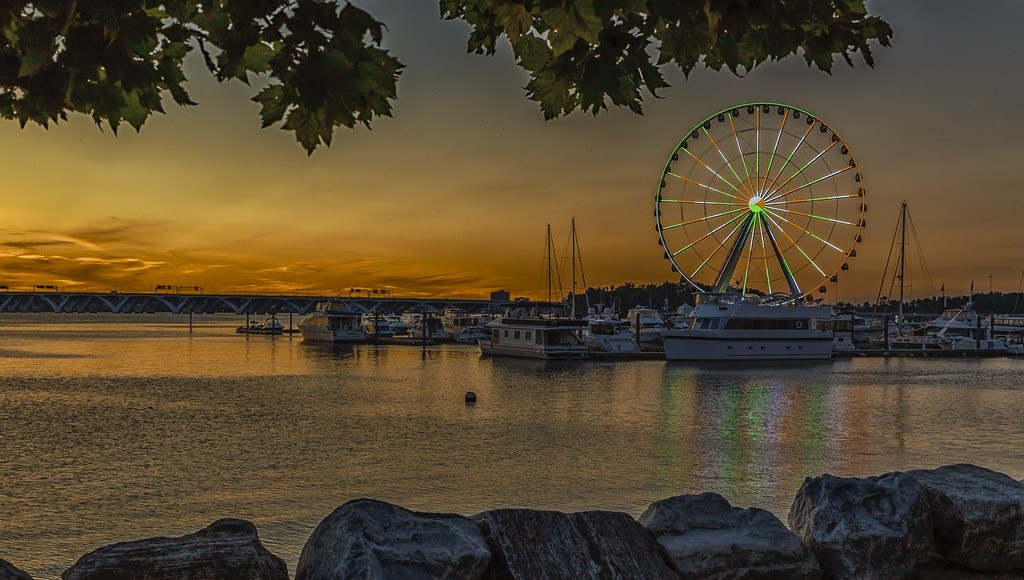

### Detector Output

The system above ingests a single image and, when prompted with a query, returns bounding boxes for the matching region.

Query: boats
[661,292,835,360]
[816,308,1024,355]
[297,216,692,360]
[237,318,285,333]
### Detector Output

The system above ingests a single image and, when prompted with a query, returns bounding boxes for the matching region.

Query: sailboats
[852,198,943,346]
